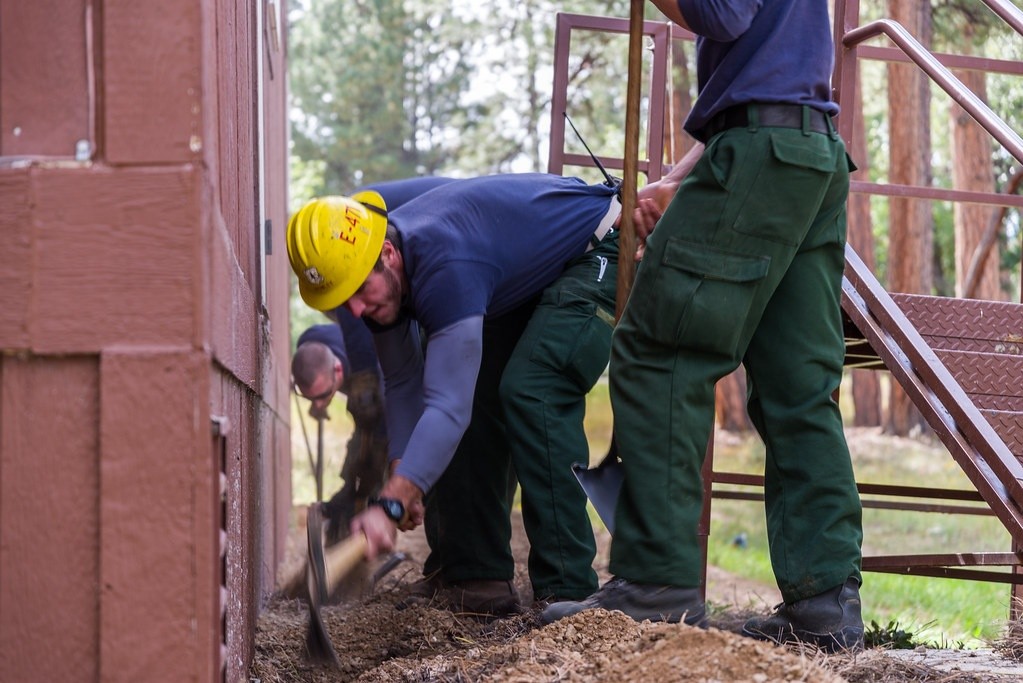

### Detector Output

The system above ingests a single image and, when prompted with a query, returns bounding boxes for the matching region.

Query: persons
[286,172,641,626]
[542,0,866,654]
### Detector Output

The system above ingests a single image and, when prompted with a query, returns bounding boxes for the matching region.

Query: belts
[584,193,622,254]
[703,103,836,145]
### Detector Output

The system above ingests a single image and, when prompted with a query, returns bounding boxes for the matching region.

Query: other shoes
[479,596,585,638]
[396,577,520,623]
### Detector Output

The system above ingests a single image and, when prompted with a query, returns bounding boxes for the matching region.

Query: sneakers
[540,575,709,629]
[741,575,864,654]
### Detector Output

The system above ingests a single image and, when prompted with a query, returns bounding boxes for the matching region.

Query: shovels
[569,0,646,541]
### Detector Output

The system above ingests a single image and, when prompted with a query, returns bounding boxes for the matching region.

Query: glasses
[293,360,335,401]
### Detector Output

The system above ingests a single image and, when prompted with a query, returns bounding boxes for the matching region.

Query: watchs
[368,495,406,521]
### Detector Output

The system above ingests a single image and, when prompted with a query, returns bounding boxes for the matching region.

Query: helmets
[286,190,388,312]
